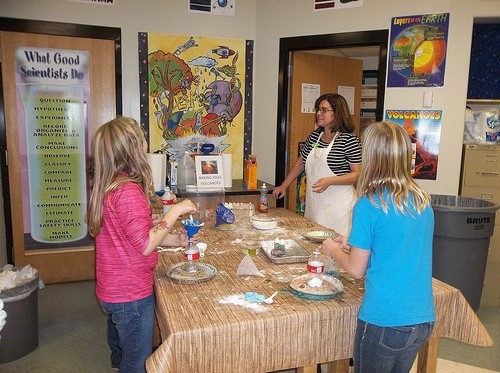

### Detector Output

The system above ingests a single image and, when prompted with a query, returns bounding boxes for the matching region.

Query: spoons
[196,243,207,257]
[264,292,278,304]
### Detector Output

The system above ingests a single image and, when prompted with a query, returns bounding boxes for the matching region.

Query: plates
[166,261,217,283]
[290,273,344,299]
[301,230,337,244]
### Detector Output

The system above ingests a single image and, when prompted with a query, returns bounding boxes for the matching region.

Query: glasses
[315,107,334,113]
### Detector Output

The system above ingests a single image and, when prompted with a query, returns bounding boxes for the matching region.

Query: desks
[145,179,494,373]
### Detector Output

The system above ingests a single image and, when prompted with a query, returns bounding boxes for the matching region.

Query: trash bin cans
[0,265,39,365]
[430,193,500,313]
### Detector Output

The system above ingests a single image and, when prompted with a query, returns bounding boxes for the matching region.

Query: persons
[322,122,436,373]
[84,117,198,373]
[272,93,362,237]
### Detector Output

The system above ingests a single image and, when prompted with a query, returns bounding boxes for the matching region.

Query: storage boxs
[244,159,258,190]
[459,142,500,227]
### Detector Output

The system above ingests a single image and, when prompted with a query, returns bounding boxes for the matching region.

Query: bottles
[307,249,325,289]
[161,187,174,213]
[177,151,196,189]
[185,238,200,277]
[274,237,285,257]
[259,183,268,213]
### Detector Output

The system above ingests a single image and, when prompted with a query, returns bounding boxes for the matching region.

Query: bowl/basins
[252,215,279,230]
